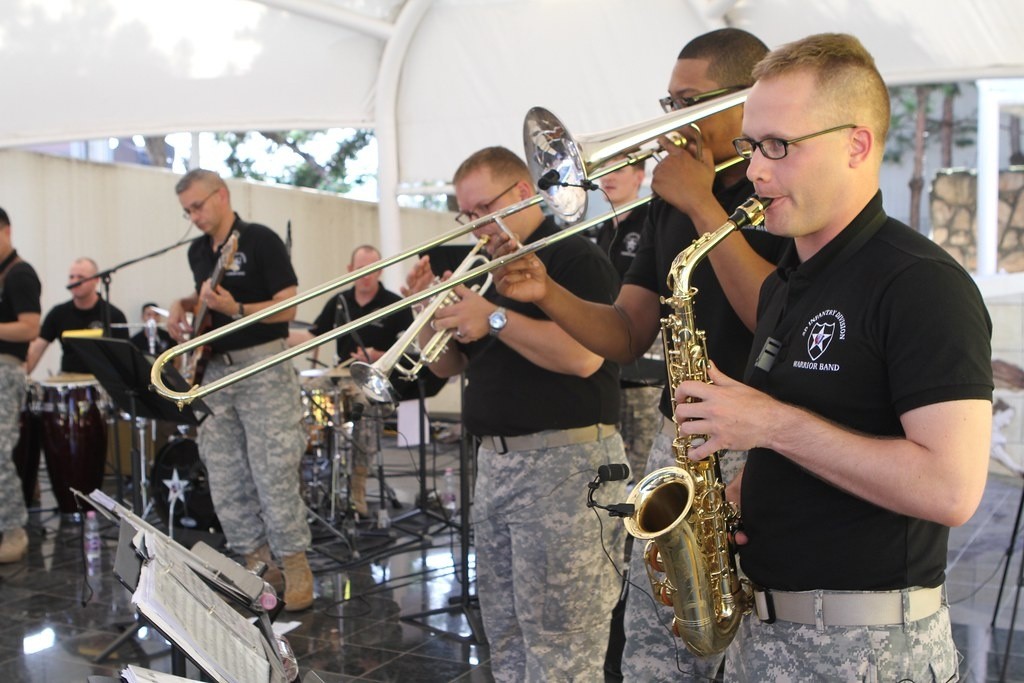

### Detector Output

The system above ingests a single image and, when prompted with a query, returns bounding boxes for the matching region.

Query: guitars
[171,227,240,387]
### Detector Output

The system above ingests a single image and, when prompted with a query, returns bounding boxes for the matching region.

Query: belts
[755,584,942,627]
[214,337,290,366]
[481,422,616,454]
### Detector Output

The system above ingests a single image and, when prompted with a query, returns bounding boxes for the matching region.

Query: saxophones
[621,193,778,660]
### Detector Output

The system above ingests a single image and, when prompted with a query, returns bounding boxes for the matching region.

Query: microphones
[333,301,343,329]
[538,170,560,190]
[598,464,629,481]
[350,402,364,421]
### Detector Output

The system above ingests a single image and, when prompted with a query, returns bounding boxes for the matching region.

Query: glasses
[181,187,220,220]
[455,181,519,226]
[731,124,857,160]
[659,84,748,114]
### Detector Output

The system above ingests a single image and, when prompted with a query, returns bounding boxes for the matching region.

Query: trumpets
[347,232,495,404]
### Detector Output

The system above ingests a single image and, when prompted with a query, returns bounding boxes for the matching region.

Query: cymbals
[149,305,192,328]
[298,367,352,379]
[288,319,319,330]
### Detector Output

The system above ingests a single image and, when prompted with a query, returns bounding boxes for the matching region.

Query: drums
[43,371,107,430]
[149,436,228,551]
[18,375,41,428]
[297,387,347,429]
[297,444,352,547]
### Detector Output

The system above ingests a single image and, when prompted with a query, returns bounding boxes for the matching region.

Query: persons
[28,258,130,513]
[487,26,790,683]
[286,245,414,514]
[166,169,312,610]
[676,32,994,683]
[134,302,178,366]
[0,207,42,561]
[601,147,667,570]
[400,147,635,683]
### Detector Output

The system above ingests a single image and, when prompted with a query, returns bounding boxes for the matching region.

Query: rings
[456,330,464,338]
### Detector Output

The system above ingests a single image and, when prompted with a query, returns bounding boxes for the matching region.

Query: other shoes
[0,530,28,562]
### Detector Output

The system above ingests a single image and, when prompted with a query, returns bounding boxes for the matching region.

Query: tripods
[309,242,490,646]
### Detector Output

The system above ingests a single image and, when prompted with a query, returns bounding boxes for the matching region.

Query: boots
[243,544,283,597]
[348,463,369,519]
[281,553,315,612]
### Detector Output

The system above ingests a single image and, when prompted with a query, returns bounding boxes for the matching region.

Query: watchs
[488,306,507,337]
[231,303,244,319]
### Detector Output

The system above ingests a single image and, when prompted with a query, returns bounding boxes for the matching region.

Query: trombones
[147,80,756,408]
